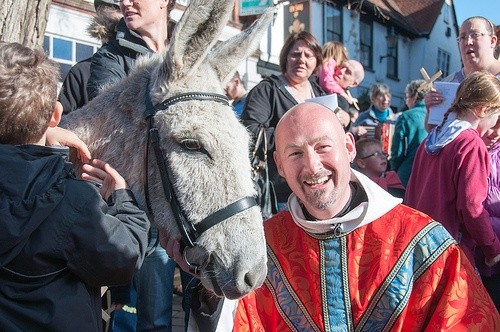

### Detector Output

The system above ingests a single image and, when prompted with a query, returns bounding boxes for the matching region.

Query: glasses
[360,151,389,161]
[455,33,491,43]
[114,0,124,2]
[403,96,410,101]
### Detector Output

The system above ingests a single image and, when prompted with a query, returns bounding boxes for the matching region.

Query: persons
[58,0,124,114]
[56,16,500,332]
[0,42,151,332]
[87,0,180,102]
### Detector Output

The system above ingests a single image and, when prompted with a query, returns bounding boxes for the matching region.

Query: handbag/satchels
[252,126,278,218]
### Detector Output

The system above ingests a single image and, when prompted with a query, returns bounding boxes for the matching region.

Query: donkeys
[47,0,280,308]
